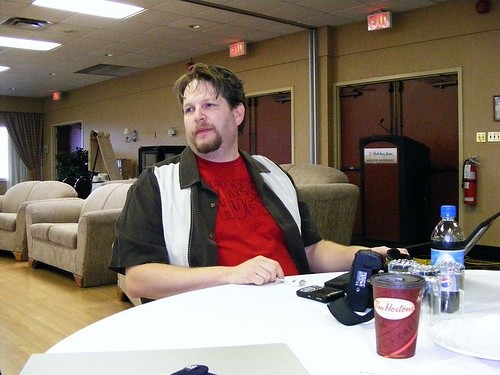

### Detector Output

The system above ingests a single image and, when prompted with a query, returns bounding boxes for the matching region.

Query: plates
[431,315,500,362]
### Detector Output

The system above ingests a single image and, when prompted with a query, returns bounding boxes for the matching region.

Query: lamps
[123,128,136,143]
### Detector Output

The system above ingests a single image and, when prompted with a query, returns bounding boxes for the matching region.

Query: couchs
[25,183,133,288]
[0,180,78,262]
[117,164,358,306]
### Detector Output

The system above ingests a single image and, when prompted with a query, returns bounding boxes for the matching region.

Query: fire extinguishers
[461,156,480,205]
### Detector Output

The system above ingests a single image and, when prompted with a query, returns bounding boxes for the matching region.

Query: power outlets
[476,132,486,142]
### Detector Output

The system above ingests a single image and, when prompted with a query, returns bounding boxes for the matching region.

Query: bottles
[430,204,466,312]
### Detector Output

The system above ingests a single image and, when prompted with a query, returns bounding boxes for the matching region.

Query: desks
[17,270,500,375]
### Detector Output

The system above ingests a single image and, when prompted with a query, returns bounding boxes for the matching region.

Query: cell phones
[296,285,344,304]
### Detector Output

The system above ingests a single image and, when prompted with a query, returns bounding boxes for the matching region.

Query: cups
[388,258,420,275]
[371,271,428,357]
[409,264,440,321]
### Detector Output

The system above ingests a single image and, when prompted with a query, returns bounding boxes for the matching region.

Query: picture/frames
[493,95,500,122]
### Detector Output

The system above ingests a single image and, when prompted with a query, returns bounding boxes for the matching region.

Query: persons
[107,63,410,305]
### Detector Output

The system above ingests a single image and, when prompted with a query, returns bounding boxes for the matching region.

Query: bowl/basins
[98,173,108,180]
[93,176,100,180]
